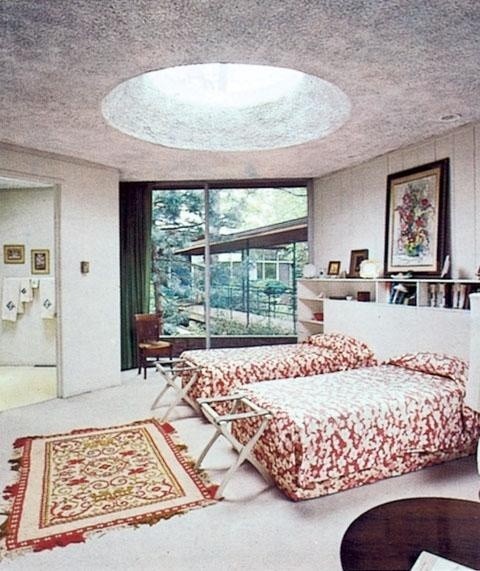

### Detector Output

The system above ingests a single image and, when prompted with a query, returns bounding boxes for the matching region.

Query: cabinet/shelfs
[295,278,480,345]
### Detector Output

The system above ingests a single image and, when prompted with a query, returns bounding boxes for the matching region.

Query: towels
[1,277,57,323]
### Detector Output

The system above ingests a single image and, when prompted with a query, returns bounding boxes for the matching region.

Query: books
[385,282,477,310]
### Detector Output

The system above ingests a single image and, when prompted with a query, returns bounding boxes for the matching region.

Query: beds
[229,349,480,502]
[179,332,378,417]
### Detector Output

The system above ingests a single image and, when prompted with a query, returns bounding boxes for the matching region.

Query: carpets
[1,418,225,562]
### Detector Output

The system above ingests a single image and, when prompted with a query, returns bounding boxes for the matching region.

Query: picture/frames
[327,249,369,279]
[384,158,450,279]
[30,250,50,275]
[3,244,25,265]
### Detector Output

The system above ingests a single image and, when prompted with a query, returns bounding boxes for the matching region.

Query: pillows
[381,351,471,384]
[303,334,372,355]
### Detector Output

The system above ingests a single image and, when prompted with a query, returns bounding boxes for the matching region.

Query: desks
[339,498,480,571]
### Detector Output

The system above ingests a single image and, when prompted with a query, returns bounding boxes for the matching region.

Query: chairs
[133,312,175,381]
